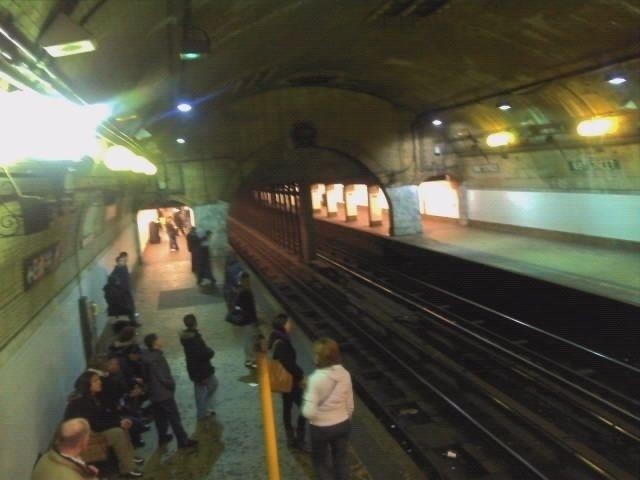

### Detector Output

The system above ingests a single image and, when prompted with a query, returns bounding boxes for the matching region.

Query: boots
[285,427,312,452]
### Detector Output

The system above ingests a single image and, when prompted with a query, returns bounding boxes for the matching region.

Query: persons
[148,206,260,324]
[299,336,355,480]
[26,248,191,480]
[179,311,219,421]
[268,313,313,453]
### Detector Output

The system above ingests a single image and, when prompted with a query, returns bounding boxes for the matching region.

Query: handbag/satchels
[267,339,293,393]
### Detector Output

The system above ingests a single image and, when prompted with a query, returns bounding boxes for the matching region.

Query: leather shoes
[135,410,217,448]
[133,456,145,463]
[120,469,143,477]
